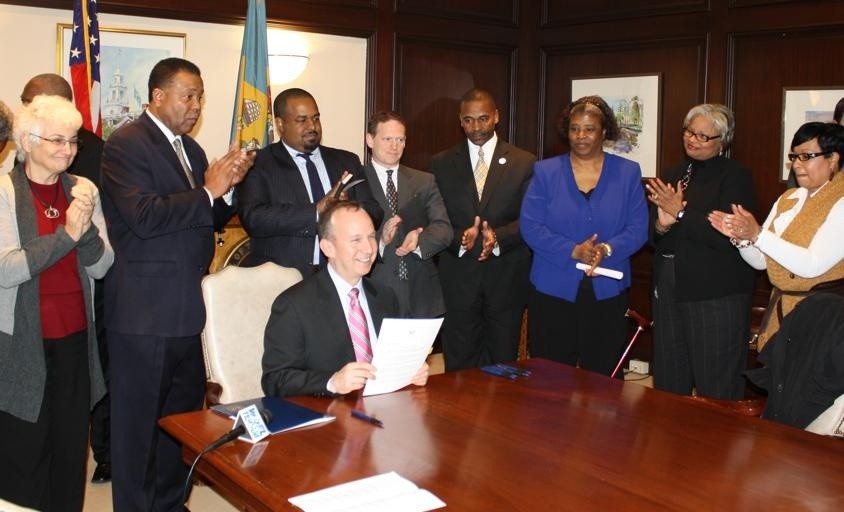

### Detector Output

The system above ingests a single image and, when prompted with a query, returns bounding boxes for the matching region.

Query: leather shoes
[92,461,112,483]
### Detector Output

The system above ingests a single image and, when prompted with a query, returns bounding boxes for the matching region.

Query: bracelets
[729,238,755,249]
[601,242,613,258]
[751,227,764,245]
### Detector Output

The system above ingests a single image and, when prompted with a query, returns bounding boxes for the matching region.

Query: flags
[229,0,274,156]
[69,0,103,140]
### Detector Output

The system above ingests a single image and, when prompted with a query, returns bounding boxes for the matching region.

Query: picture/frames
[56,23,187,142]
[570,73,663,182]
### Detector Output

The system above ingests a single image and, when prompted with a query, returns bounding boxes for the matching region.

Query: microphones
[203,403,273,451]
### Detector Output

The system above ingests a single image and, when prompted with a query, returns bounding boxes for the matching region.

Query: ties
[297,153,328,270]
[474,149,490,202]
[174,139,198,189]
[347,287,373,363]
[386,169,409,282]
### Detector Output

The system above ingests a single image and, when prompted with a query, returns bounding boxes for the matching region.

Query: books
[335,173,353,198]
[209,395,336,436]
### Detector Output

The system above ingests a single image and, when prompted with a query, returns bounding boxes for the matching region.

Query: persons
[785,99,844,189]
[0,98,14,153]
[21,74,111,484]
[261,200,430,398]
[425,87,538,374]
[0,94,116,512]
[236,88,385,280]
[519,95,650,380]
[645,103,755,401]
[362,110,454,354]
[97,58,258,511]
[707,122,844,365]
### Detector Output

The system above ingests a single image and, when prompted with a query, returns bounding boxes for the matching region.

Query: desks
[155,356,844,511]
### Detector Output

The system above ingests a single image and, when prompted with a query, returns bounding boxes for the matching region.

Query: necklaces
[25,175,60,219]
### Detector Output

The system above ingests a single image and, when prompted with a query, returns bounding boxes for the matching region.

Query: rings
[652,192,658,199]
[740,226,743,233]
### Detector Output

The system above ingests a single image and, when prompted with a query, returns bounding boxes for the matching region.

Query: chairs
[200,262,305,409]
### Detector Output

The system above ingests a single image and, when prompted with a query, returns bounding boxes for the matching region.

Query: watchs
[493,232,499,248]
[676,209,686,221]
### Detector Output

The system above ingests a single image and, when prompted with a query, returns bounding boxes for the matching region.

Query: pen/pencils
[481,362,532,379]
[351,410,384,427]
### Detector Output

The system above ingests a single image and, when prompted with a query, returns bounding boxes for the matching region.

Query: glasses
[29,131,83,150]
[680,125,722,143]
[788,151,830,162]
